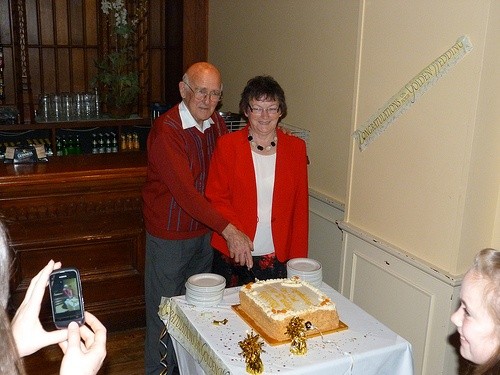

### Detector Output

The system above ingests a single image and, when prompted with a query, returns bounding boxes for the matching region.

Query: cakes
[238,274,339,341]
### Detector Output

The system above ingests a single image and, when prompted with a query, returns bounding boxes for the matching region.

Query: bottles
[56,134,80,157]
[152,102,160,126]
[121,132,140,151]
[91,131,118,154]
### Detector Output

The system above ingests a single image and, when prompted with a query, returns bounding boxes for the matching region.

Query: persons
[138,62,254,375]
[63,284,74,300]
[451,247,500,375]
[209,74,307,291]
[0,215,108,375]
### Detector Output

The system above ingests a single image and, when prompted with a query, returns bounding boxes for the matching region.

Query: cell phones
[48,267,85,329]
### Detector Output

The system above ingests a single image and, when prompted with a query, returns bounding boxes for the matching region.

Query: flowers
[93,1,150,100]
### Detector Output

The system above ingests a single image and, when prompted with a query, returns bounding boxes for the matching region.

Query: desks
[156,279,416,375]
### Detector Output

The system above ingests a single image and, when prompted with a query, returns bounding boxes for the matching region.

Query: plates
[287,258,323,288]
[184,273,226,308]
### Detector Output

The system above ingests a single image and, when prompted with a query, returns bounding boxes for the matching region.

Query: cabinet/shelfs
[0,115,148,331]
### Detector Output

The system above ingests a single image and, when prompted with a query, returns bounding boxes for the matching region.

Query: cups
[40,92,96,116]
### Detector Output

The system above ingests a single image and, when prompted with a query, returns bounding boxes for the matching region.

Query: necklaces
[248,132,277,152]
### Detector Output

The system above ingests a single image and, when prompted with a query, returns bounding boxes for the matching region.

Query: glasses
[249,103,281,114]
[185,83,223,102]
